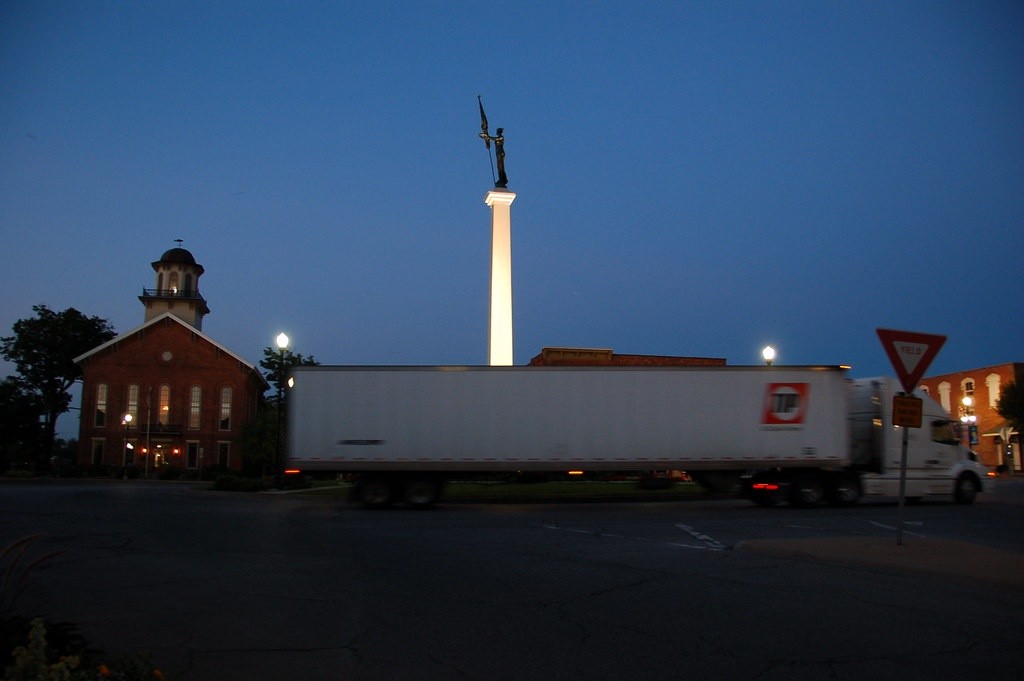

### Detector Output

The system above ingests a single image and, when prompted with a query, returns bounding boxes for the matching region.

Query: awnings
[982,419,1019,436]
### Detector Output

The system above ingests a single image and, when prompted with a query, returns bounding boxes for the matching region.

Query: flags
[480,101,491,149]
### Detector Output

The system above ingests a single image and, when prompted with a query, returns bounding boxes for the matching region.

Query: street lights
[123,413,133,481]
[272,331,291,461]
[960,396,976,450]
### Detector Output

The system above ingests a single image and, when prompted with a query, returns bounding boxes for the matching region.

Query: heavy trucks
[285,364,997,512]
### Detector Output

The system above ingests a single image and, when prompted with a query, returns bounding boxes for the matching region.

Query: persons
[480,128,508,186]
